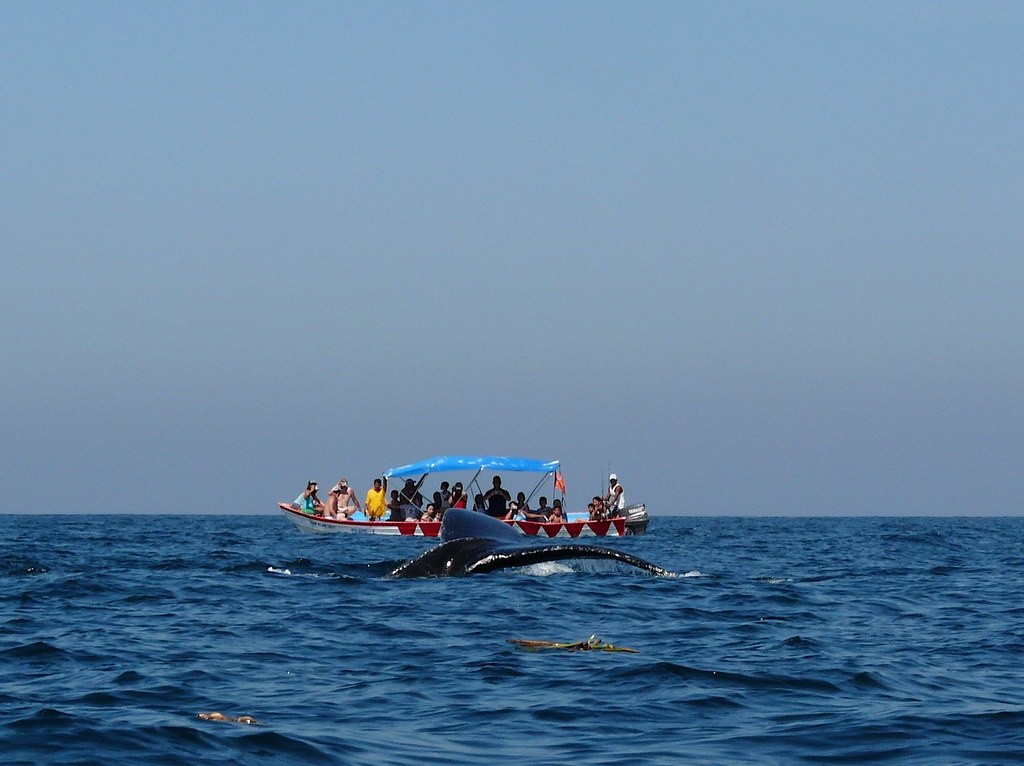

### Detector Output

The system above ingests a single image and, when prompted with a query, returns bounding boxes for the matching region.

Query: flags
[555,471,566,495]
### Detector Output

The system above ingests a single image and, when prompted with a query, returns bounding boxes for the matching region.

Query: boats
[278,456,650,538]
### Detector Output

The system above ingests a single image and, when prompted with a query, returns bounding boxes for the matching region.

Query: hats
[309,480,317,484]
[405,478,416,485]
[610,474,617,480]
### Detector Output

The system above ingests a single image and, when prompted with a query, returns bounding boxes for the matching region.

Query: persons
[364,473,429,521]
[588,473,627,521]
[473,476,563,523]
[421,481,468,522]
[291,478,365,521]
